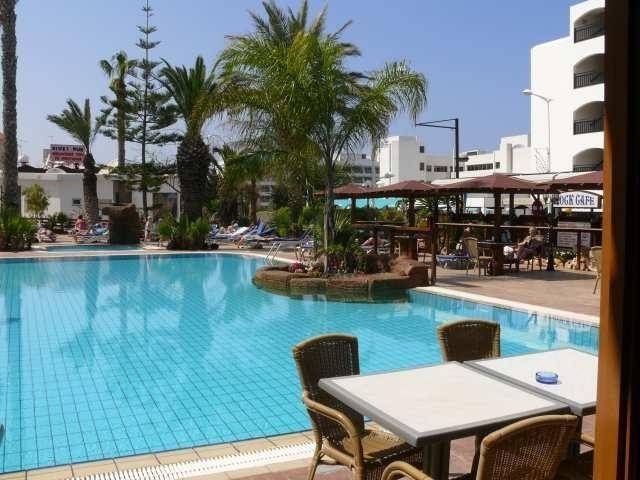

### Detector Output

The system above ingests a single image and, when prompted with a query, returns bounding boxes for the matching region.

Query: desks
[319,360,571,479]
[464,346,600,453]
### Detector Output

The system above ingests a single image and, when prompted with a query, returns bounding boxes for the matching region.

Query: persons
[217,222,239,234]
[513,226,546,264]
[143,215,153,242]
[236,229,258,248]
[358,232,391,255]
[72,213,108,237]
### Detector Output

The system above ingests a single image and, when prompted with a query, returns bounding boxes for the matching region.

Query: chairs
[356,236,543,277]
[73,225,316,259]
[382,460,431,479]
[452,415,579,480]
[590,246,601,294]
[292,332,417,479]
[436,318,501,361]
